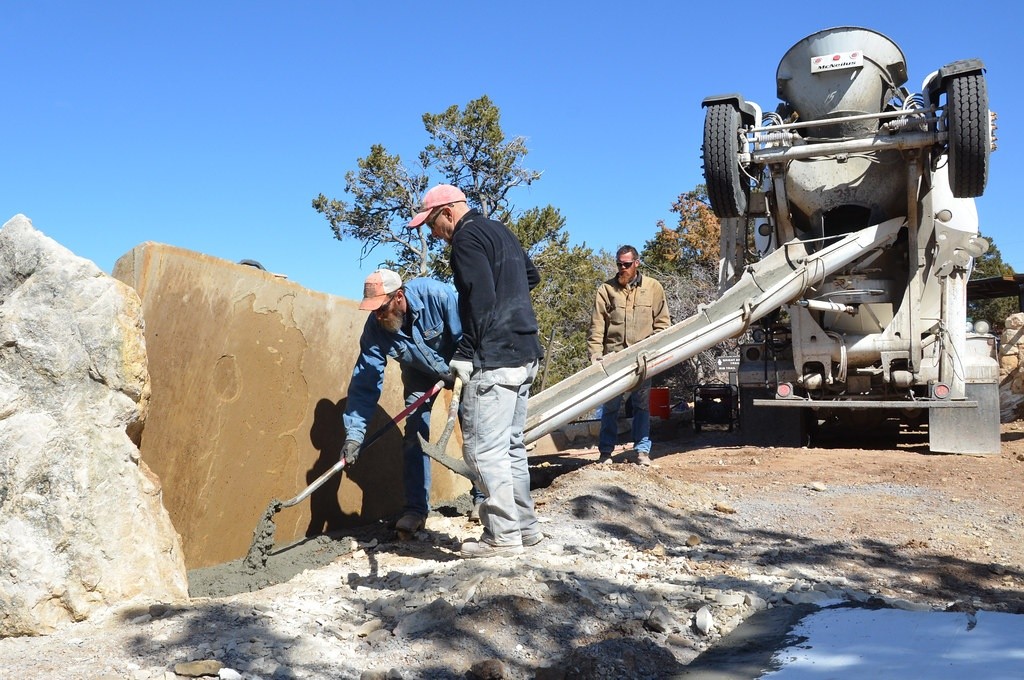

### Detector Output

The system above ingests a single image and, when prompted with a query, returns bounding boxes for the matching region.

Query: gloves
[439,370,456,390]
[448,359,473,385]
[339,440,361,468]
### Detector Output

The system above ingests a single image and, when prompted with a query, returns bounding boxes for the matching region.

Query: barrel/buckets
[649,387,670,419]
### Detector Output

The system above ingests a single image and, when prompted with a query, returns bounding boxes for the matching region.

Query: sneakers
[599,453,613,464]
[461,540,524,558]
[470,496,484,520]
[521,532,544,546]
[634,452,650,466]
[396,514,425,532]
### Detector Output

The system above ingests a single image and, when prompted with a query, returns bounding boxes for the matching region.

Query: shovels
[416,375,478,483]
[242,381,446,562]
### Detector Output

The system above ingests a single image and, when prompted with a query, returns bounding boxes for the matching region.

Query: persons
[587,245,671,465]
[339,183,545,558]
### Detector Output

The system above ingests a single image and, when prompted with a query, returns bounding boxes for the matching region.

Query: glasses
[372,294,396,312]
[427,209,444,230]
[617,261,635,267]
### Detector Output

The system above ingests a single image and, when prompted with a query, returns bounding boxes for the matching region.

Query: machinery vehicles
[526,18,1005,456]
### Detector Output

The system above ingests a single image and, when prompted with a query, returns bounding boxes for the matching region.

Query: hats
[359,269,402,311]
[409,184,467,227]
